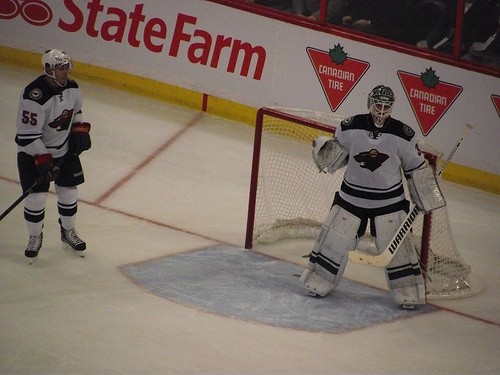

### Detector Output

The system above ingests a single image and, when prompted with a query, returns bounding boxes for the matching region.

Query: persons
[299,85,447,309]
[255,0,500,67]
[15,49,92,265]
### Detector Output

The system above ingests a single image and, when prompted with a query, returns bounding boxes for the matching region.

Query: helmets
[41,49,71,79]
[367,85,395,128]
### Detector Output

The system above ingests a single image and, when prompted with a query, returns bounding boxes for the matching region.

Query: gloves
[33,153,56,182]
[69,122,91,155]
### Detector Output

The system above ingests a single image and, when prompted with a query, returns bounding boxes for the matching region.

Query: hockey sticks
[0,147,76,221]
[348,123,473,266]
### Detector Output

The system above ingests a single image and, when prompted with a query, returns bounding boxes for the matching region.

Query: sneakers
[60,226,86,257]
[24,232,44,265]
